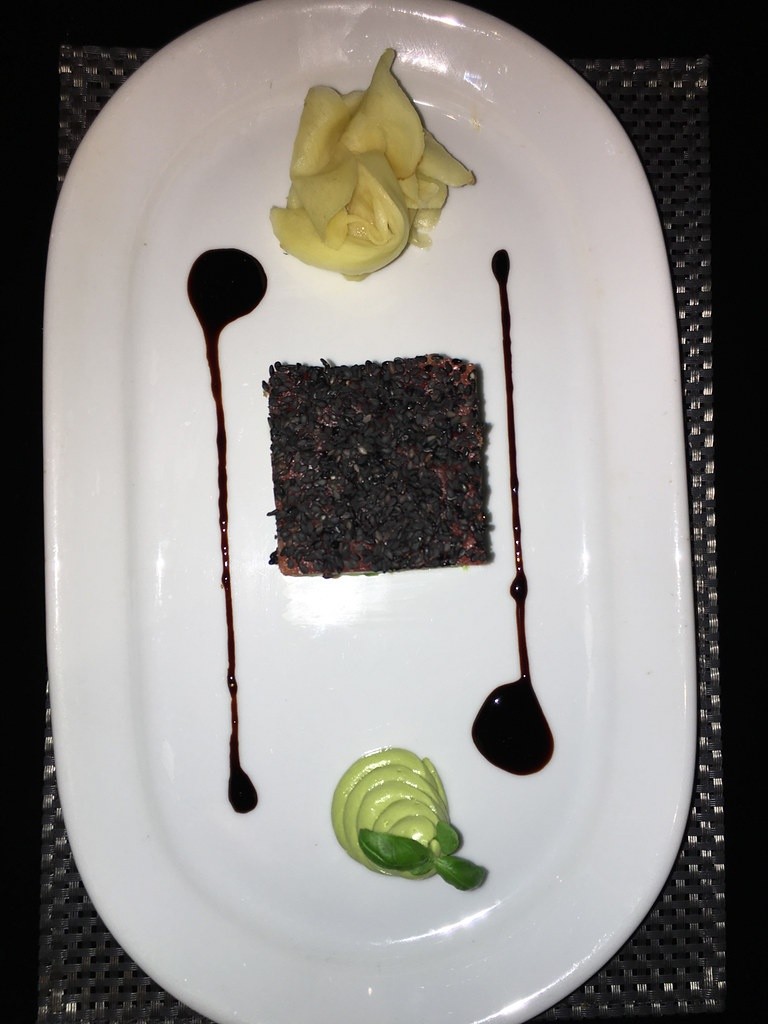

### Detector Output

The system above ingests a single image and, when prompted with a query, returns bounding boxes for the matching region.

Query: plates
[45,0,694,1024]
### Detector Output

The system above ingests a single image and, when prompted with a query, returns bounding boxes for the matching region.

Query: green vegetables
[358,822,487,892]
